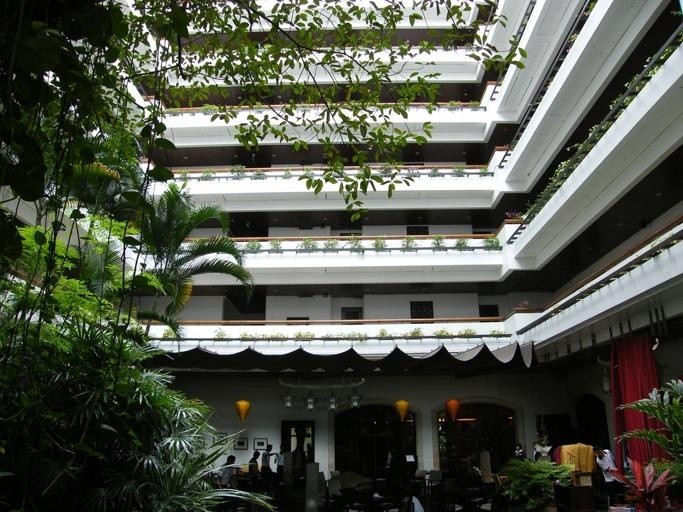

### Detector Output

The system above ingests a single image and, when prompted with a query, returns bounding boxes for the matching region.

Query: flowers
[504,209,522,219]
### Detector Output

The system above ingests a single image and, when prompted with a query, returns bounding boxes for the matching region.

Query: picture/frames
[211,432,268,451]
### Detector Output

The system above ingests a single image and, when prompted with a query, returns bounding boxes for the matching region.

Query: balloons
[443,398,462,422]
[234,399,250,422]
[393,399,410,422]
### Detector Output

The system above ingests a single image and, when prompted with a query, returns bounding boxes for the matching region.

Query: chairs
[317,467,498,511]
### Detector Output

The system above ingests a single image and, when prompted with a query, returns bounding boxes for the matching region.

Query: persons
[261,443,276,472]
[514,442,527,460]
[248,450,260,473]
[531,440,540,462]
[272,442,286,474]
[591,443,625,506]
[217,454,236,511]
[306,442,312,459]
